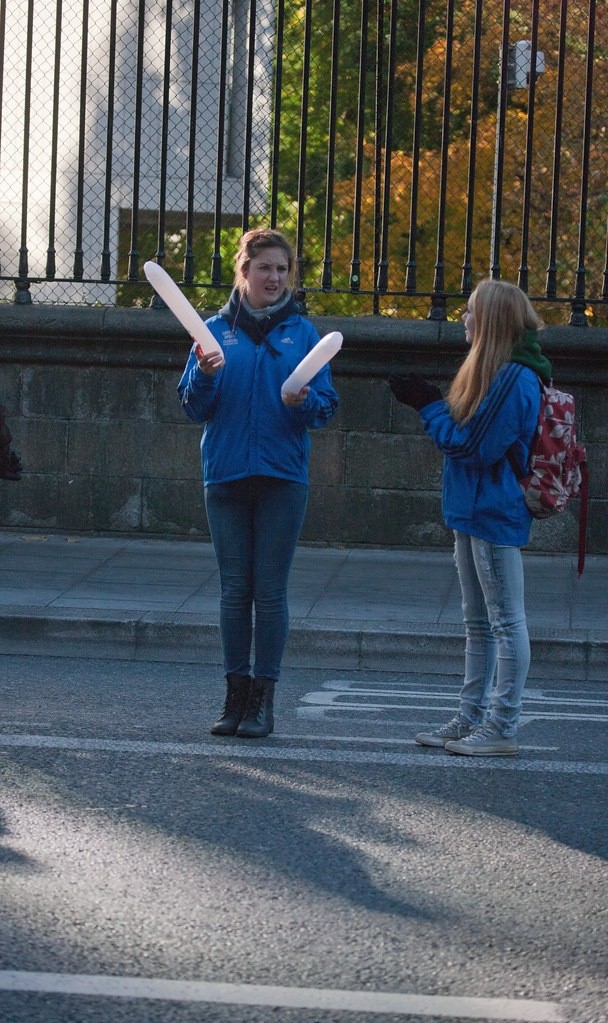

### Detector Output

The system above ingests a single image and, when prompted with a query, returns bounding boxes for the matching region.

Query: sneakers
[444,719,518,755]
[415,714,477,746]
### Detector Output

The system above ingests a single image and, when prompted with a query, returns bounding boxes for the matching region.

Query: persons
[177,229,341,739]
[387,280,551,757]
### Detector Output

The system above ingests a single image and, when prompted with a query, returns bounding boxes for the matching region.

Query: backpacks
[505,368,588,579]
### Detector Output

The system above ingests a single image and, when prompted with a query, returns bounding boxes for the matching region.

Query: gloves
[388,372,443,412]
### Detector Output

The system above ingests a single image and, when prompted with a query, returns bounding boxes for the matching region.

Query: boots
[210,675,250,734]
[237,677,274,737]
